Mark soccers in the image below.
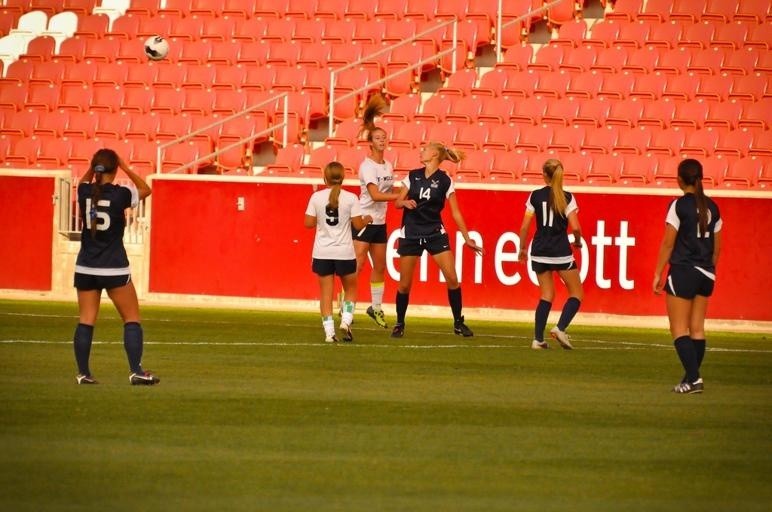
[144,36,171,61]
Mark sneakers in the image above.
[550,326,572,349]
[339,309,353,342]
[75,374,98,385]
[671,378,705,393]
[325,335,339,343]
[392,323,404,336]
[367,306,387,328]
[128,370,160,386]
[531,340,551,350]
[454,316,473,337]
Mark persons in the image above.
[392,141,486,337]
[338,127,400,328]
[652,159,723,395]
[518,159,583,351]
[72,148,160,385]
[302,162,373,342]
